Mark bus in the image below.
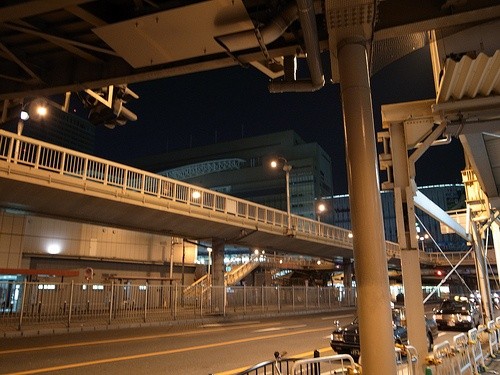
[331,272,357,288]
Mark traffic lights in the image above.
[436,268,443,276]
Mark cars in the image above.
[330,301,439,361]
[471,288,500,309]
[434,296,479,330]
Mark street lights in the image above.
[317,201,325,221]
[206,247,212,284]
[271,157,292,231]
[417,231,429,251]
[13,98,48,163]
